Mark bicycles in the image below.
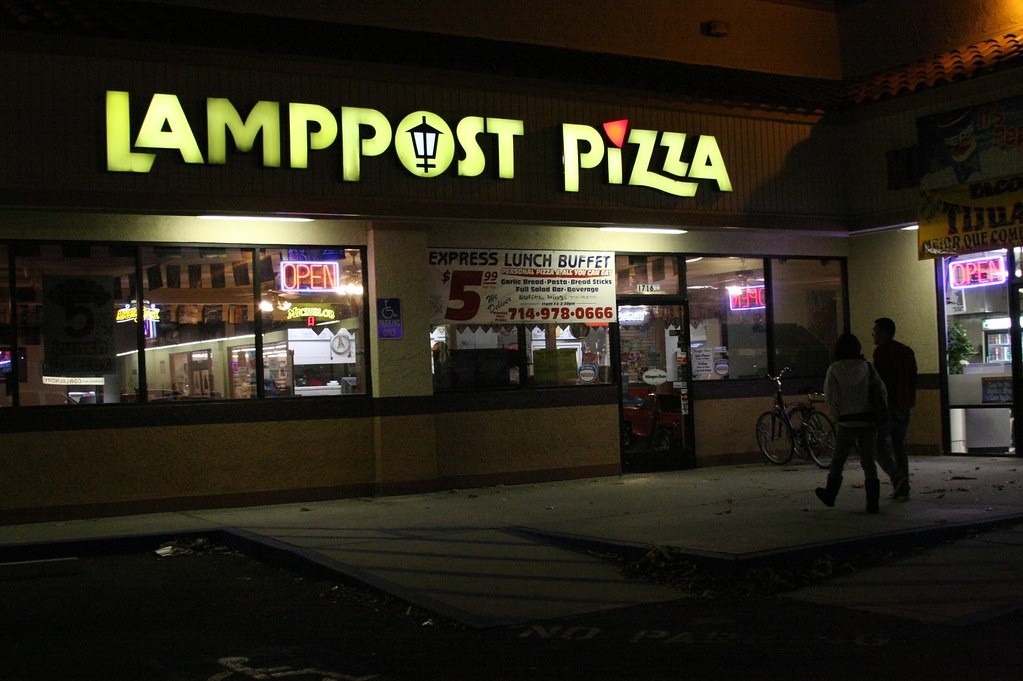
[753,366,859,470]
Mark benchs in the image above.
[625,407,681,453]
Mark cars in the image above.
[623,390,682,451]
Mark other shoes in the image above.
[889,486,912,501]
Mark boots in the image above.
[814,472,844,506]
[864,478,880,515]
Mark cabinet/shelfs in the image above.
[270,368,289,391]
[233,371,256,398]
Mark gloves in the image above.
[878,408,891,430]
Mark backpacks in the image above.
[828,359,876,405]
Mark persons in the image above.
[815,332,887,513]
[871,317,917,501]
